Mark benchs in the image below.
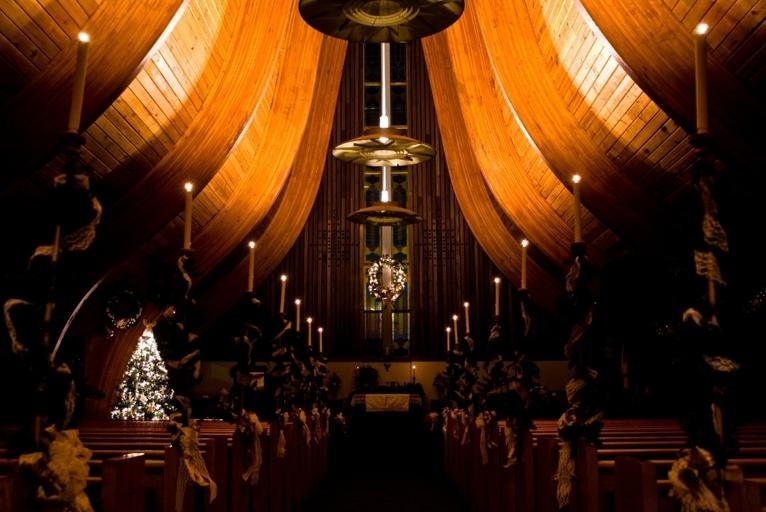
[0,407,330,512]
[441,408,766,511]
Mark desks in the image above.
[351,387,424,407]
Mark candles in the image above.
[279,274,324,351]
[184,183,192,248]
[522,238,529,287]
[493,277,502,316]
[247,240,255,292]
[444,301,470,352]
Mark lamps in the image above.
[297,0,467,229]
